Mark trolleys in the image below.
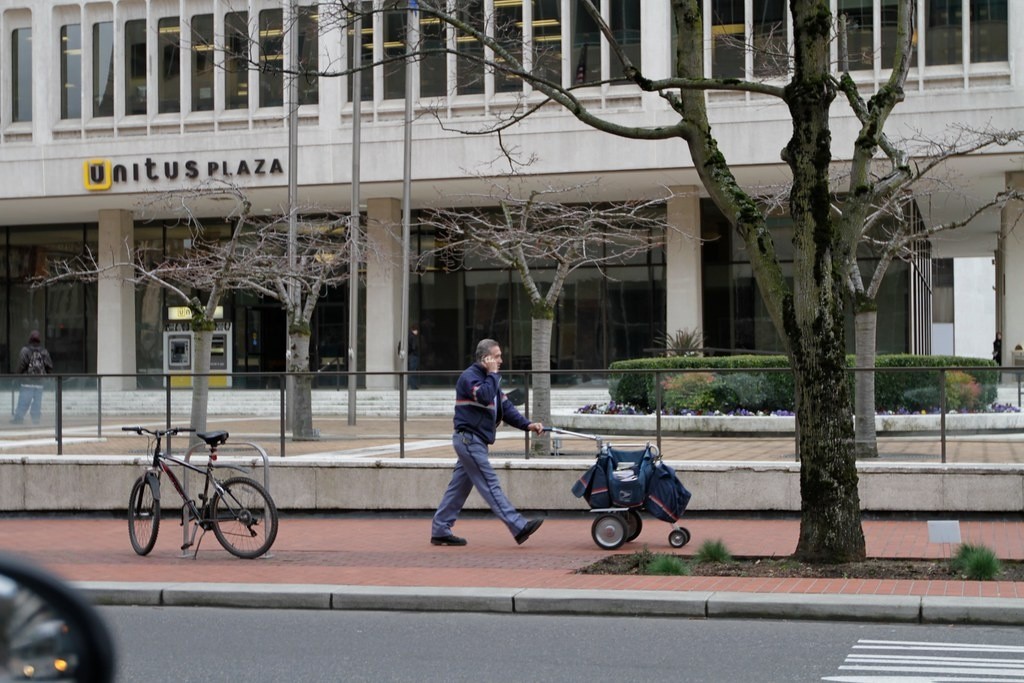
[543,424,692,551]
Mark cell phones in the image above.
[481,358,485,363]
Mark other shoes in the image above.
[33,421,40,425]
[9,418,23,424]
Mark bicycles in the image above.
[121,425,279,563]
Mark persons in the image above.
[992,332,1002,384]
[9,331,54,424]
[409,328,420,390]
[430,339,544,547]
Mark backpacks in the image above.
[24,345,48,380]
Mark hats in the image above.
[29,330,40,339]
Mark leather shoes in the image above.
[431,534,468,546]
[514,519,544,545]
